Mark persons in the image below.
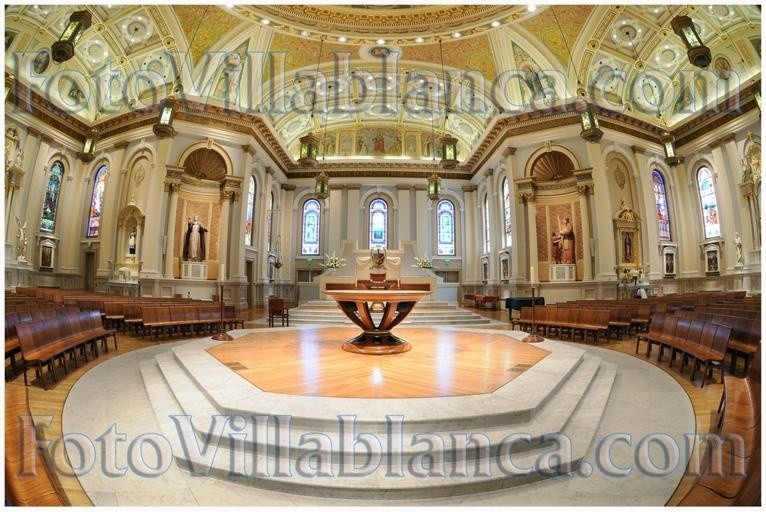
[551,231,563,264]
[356,130,405,156]
[187,215,209,262]
[127,225,138,254]
[560,216,575,263]
[624,232,632,260]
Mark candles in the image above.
[530,266,534,286]
[220,264,225,282]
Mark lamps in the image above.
[152,4,213,139]
[546,5,606,146]
[426,173,442,202]
[659,5,712,70]
[622,27,680,164]
[433,37,458,169]
[80,26,142,163]
[314,171,330,201]
[296,36,325,166]
[51,7,93,63]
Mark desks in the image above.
[464,294,500,310]
[320,281,431,354]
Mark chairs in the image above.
[266,297,290,327]
[510,285,761,507]
[6,288,244,506]
